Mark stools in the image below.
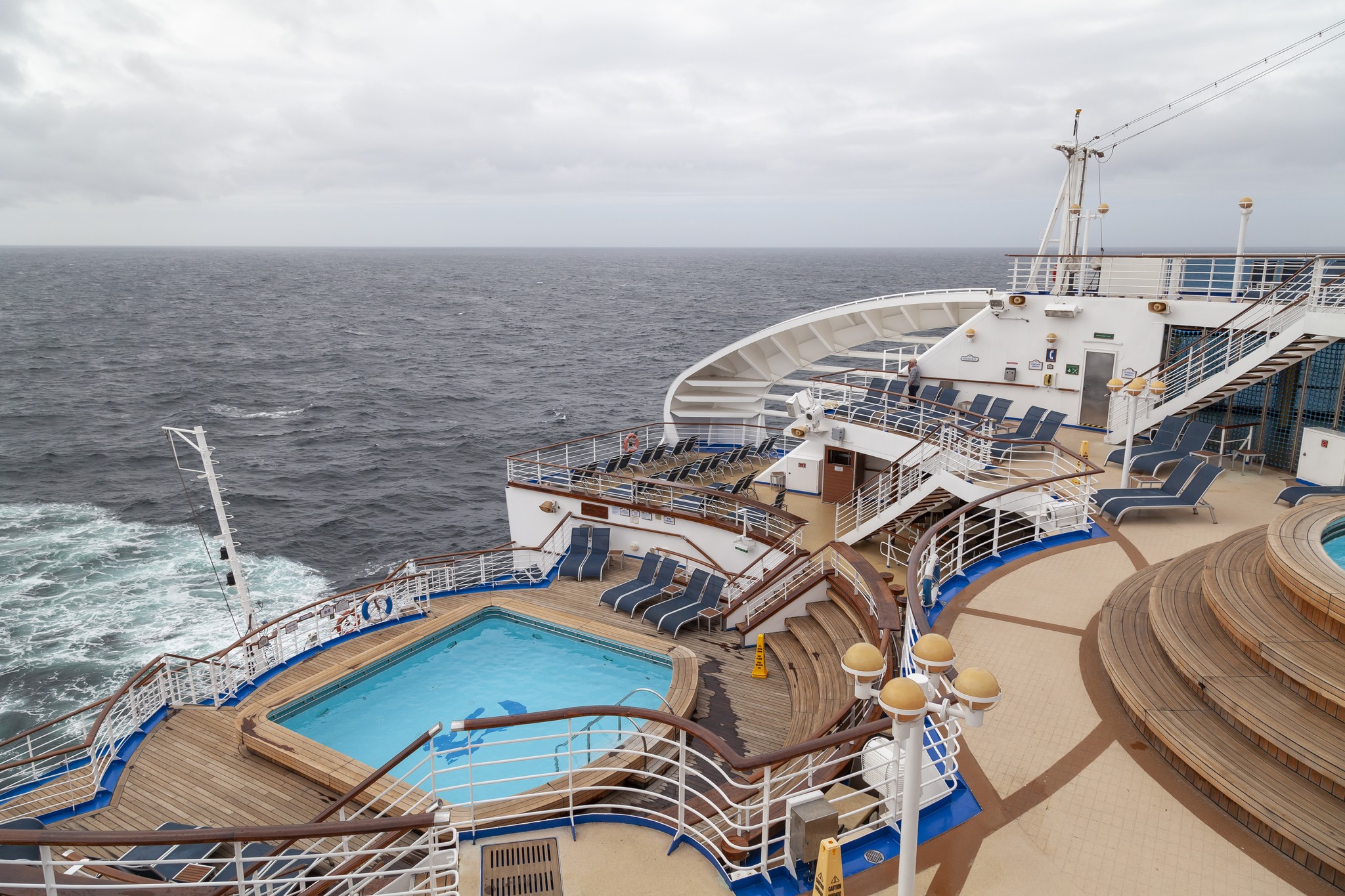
[720,836,748,878]
[888,584,905,613]
[896,597,907,642]
[879,572,894,587]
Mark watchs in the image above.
[908,384,911,386]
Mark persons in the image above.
[908,358,921,409]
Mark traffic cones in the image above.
[752,632,769,678]
[812,837,844,896]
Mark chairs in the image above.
[1273,486,1345,506]
[849,757,879,829]
[598,552,727,639]
[558,527,590,582]
[1089,415,1225,526]
[82,822,334,896]
[580,527,610,582]
[537,435,788,529]
[513,563,543,583]
[833,378,1069,464]
[1241,260,1345,306]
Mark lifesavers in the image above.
[361,593,393,624]
[1052,263,1058,283]
[624,434,639,454]
[336,610,360,636]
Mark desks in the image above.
[958,401,973,417]
[1231,449,1266,476]
[1130,476,1164,488]
[607,550,624,570]
[1189,450,1222,468]
[770,471,786,491]
[824,782,880,830]
[737,481,755,498]
[697,607,722,635]
[690,444,699,460]
[592,470,602,488]
[895,401,916,412]
[660,584,684,602]
[995,423,1019,434]
[172,863,216,883]
[769,502,788,512]
[660,452,669,468]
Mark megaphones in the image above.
[1008,295,1026,306]
[1148,300,1169,313]
